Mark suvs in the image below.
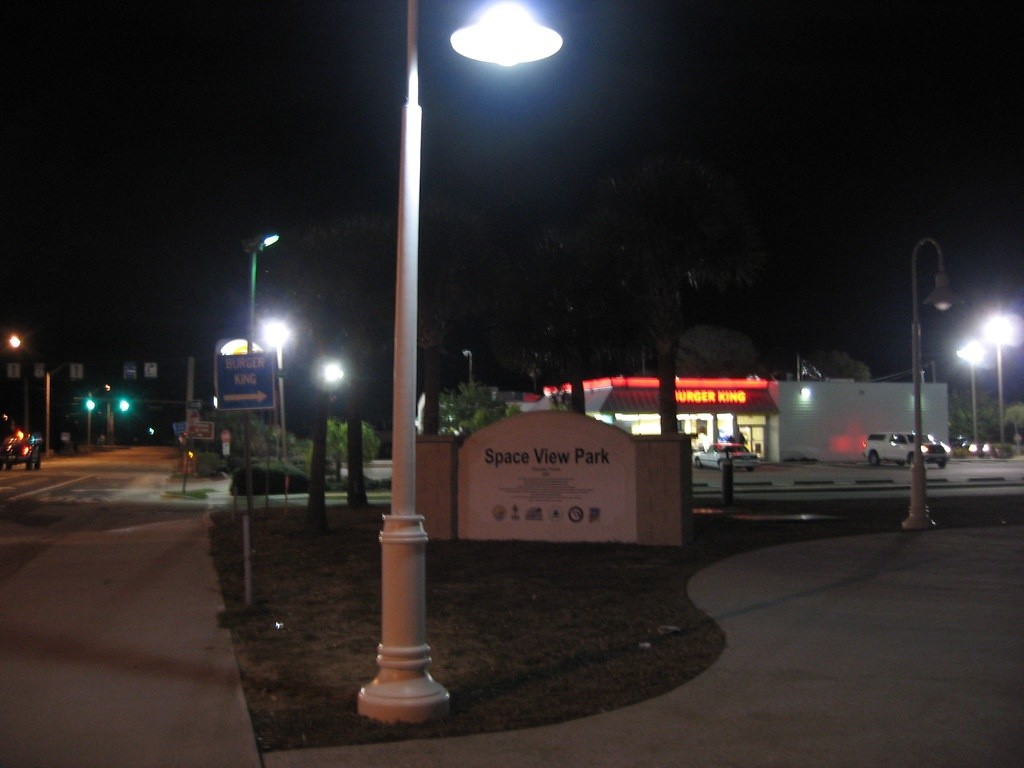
[862,432,954,468]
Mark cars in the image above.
[691,442,762,470]
[0,434,42,470]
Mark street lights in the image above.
[86,401,95,449]
[899,237,968,532]
[246,234,282,507]
[984,318,1011,445]
[962,342,985,448]
[463,350,474,386]
[354,0,562,725]
[10,335,29,432]
